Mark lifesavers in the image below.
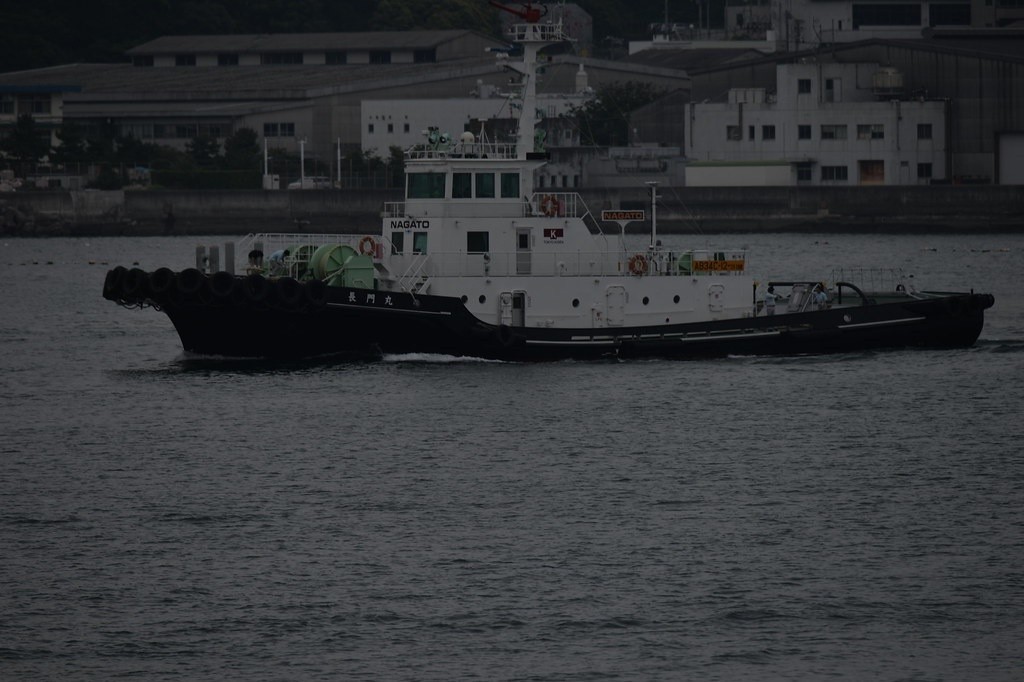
[359,236,376,256]
[631,253,647,272]
[104,266,325,310]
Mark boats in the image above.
[103,0,996,360]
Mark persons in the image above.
[814,282,827,311]
[765,287,778,316]
[249,249,290,276]
[459,127,475,153]
[541,194,560,218]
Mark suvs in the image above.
[287,176,331,191]
